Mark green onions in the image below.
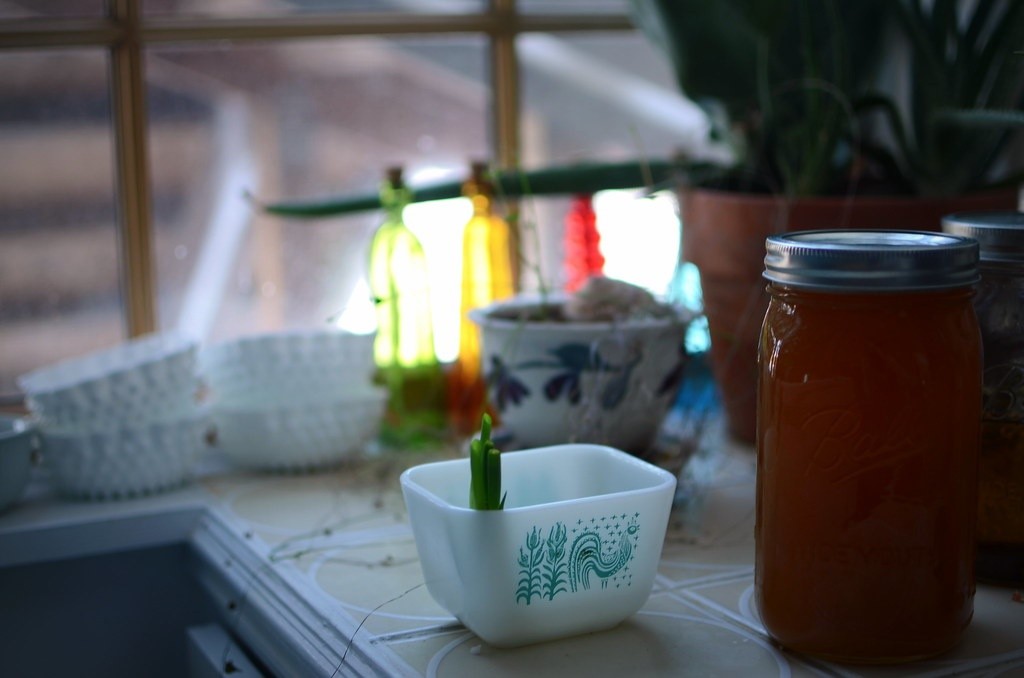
[468,412,507,510]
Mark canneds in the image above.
[752,211,1024,667]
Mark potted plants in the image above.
[623,1,1022,446]
[474,275,695,456]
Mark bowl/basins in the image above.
[0,415,33,516]
[400,442,678,649]
[19,327,387,501]
